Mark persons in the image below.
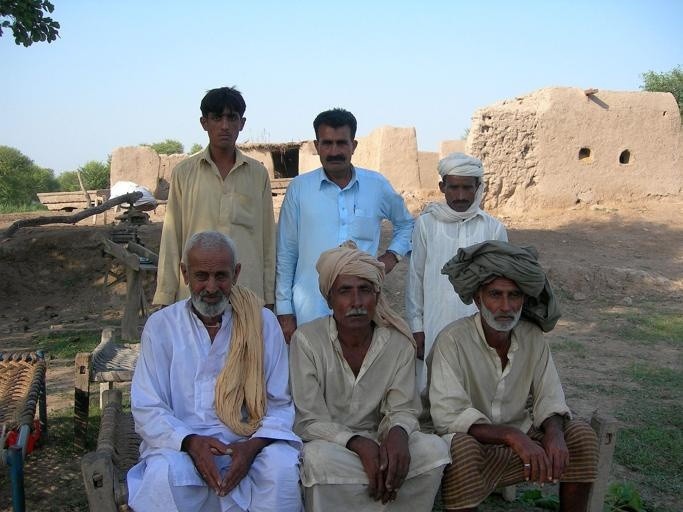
[275,109,414,345]
[407,152,508,361]
[289,240,448,512]
[131,230,302,511]
[429,241,599,512]
[152,88,277,314]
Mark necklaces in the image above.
[202,319,225,330]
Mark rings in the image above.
[523,463,531,467]
